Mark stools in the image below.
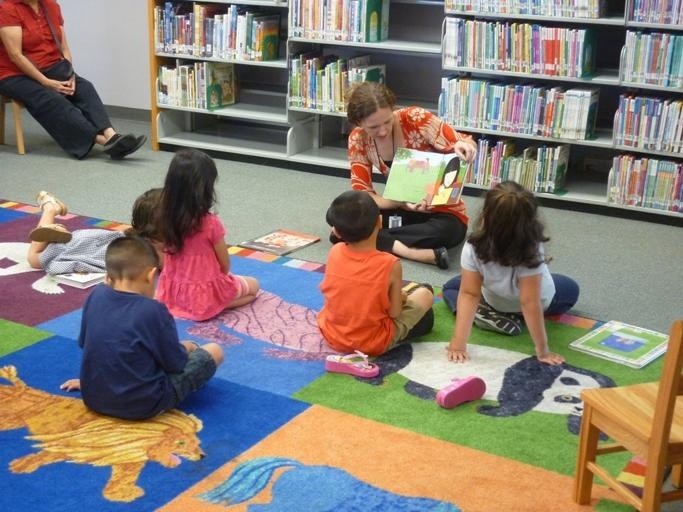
[0,96,29,155]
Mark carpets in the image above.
[0,200,682,512]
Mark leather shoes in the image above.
[330,230,339,246]
[432,250,449,268]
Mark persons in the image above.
[60,236,224,421]
[327,82,479,270]
[317,191,434,356]
[0,0,148,160]
[27,188,167,277]
[442,181,579,367]
[153,149,259,322]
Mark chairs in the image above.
[574,321,683,512]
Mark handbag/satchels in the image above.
[41,4,76,80]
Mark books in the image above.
[464,137,569,194]
[287,0,390,44]
[443,16,598,79]
[238,228,321,257]
[441,73,602,141]
[622,29,682,88]
[155,59,238,110]
[625,1,683,26]
[609,151,683,213]
[443,1,609,19]
[617,91,683,154]
[52,273,112,289]
[287,51,386,113]
[155,1,281,61]
[383,148,472,206]
[569,320,670,369]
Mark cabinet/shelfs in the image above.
[149,2,683,220]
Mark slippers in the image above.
[432,377,486,409]
[323,351,381,377]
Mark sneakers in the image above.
[104,131,135,155]
[134,137,145,154]
[470,307,519,335]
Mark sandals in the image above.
[38,192,69,218]
[29,226,70,243]
